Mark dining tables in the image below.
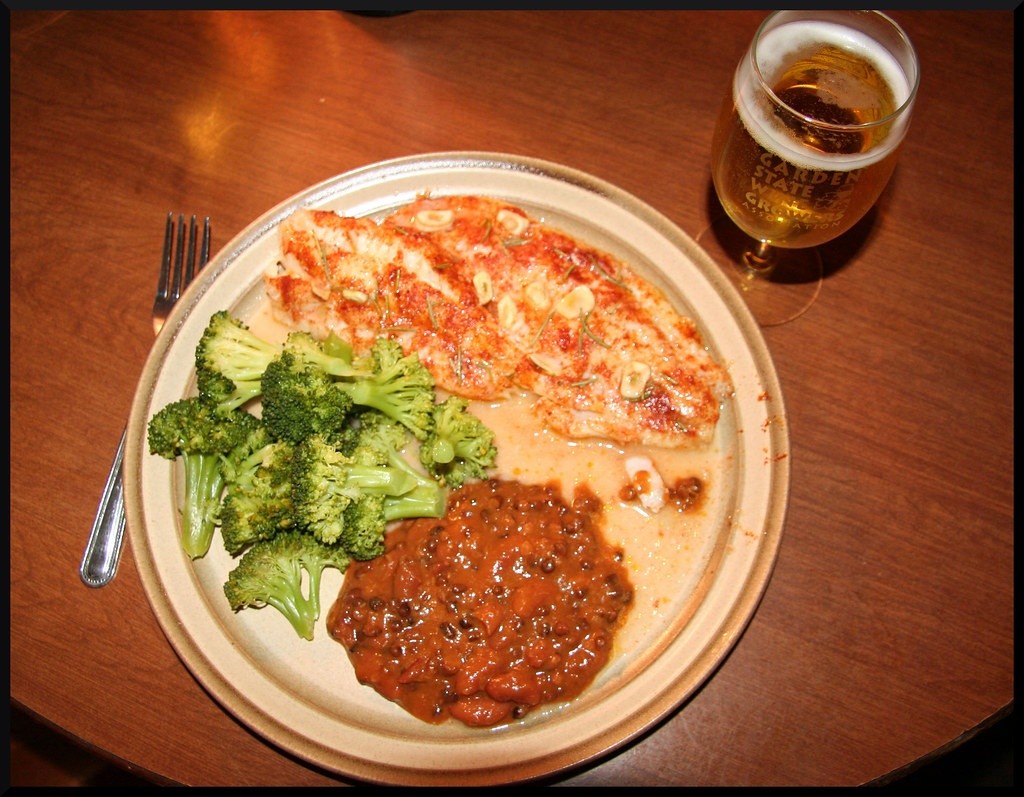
[9,10,1013,795]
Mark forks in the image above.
[78,210,212,588]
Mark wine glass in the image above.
[694,10,920,327]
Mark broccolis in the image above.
[149,314,491,638]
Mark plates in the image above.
[121,151,790,787]
